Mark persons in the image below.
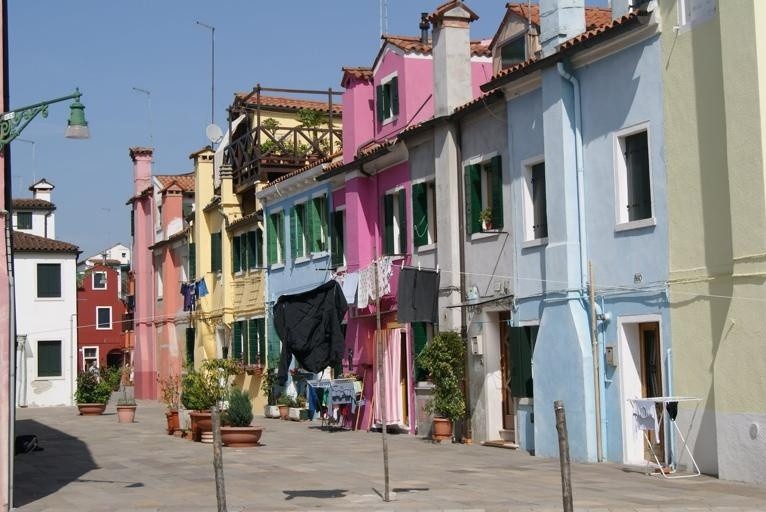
[89,361,100,383]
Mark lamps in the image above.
[1,84,88,153]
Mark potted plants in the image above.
[72,355,139,423]
[411,330,469,436]
[154,351,309,447]
